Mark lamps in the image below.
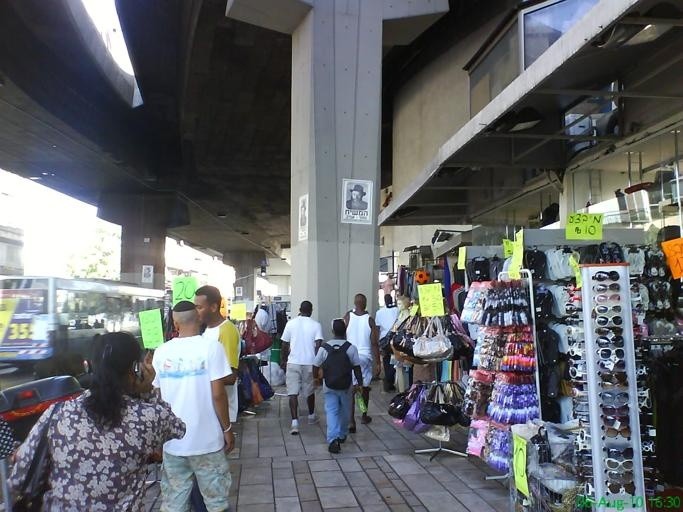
[391,206,419,218]
[597,23,674,49]
[437,167,482,177]
[485,107,542,133]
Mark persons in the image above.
[194,285,241,424]
[4,332,186,512]
[151,300,235,512]
[279,291,400,454]
[299,201,307,226]
[346,184,368,210]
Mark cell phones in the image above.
[133,360,145,381]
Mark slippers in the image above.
[536,283,574,399]
[525,247,580,280]
[631,279,677,335]
[582,241,671,279]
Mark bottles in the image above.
[356,392,367,413]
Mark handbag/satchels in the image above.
[388,383,471,442]
[239,372,276,412]
[246,330,274,356]
[377,327,475,365]
[11,439,54,512]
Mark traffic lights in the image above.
[260,265,266,277]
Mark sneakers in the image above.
[329,420,356,453]
[361,416,371,423]
[290,426,299,434]
[308,416,321,425]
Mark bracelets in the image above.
[223,425,232,434]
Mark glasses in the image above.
[563,271,656,496]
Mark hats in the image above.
[350,185,366,196]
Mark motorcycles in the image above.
[0,353,96,443]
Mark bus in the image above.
[0,274,170,366]
[259,295,290,321]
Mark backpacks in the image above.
[320,341,352,390]
